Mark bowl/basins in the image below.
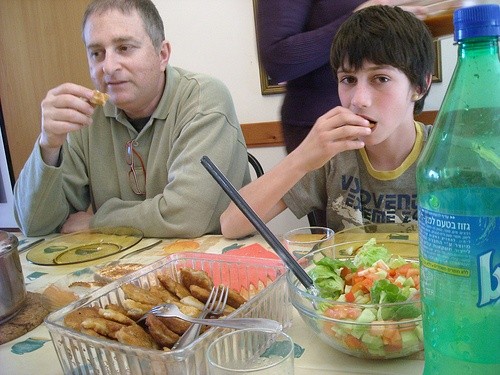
[285,241,426,358]
[46,251,289,375]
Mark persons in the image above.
[257,0,463,234]
[220,4,500,238]
[13,0,253,238]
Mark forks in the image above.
[171,281,230,358]
[134,304,283,334]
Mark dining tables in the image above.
[0,232,425,375]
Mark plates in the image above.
[26,227,145,266]
[332,220,419,256]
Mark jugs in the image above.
[0,231,26,325]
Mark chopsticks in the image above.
[198,156,315,292]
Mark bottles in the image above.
[417,5,500,375]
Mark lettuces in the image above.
[305,234,423,324]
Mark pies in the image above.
[60,266,250,354]
[88,90,110,106]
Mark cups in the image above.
[205,328,294,375]
[285,227,333,259]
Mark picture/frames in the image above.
[253,0,443,96]
